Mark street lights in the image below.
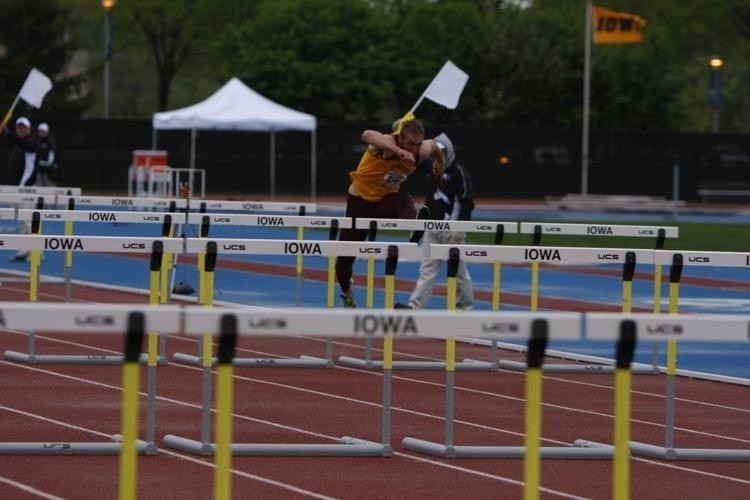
[101,0,118,118]
[709,54,725,131]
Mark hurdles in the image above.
[337,218,519,371]
[628,248,750,461]
[183,304,582,500]
[163,234,421,457]
[55,194,187,307]
[188,199,318,306]
[3,208,188,367]
[0,300,182,500]
[2,232,184,455]
[174,212,353,369]
[0,186,82,284]
[402,243,656,459]
[500,220,679,376]
[584,310,750,500]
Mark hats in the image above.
[37,122,49,132]
[15,116,30,127]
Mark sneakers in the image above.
[409,206,431,244]
[393,302,417,312]
[340,278,357,309]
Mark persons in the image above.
[5,117,39,263]
[391,132,475,314]
[36,123,60,186]
[333,118,445,308]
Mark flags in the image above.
[19,66,54,109]
[591,8,648,46]
[423,62,470,110]
